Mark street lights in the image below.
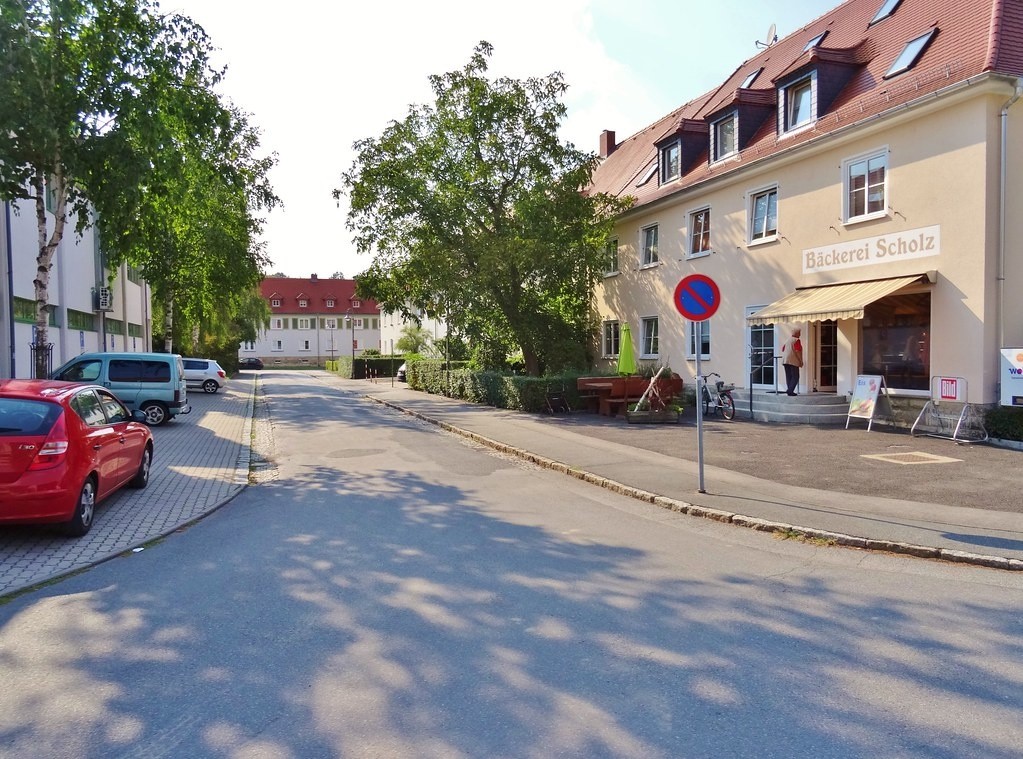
[344,308,355,378]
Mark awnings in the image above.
[746,273,926,326]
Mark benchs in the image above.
[577,377,683,418]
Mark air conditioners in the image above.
[99,286,112,310]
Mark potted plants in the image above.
[626,400,685,425]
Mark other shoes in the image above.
[786,390,797,396]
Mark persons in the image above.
[782,328,803,396]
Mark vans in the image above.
[46,353,188,426]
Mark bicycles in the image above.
[695,372,736,420]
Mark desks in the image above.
[584,382,613,415]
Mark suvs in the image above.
[181,358,227,394]
[239,358,264,370]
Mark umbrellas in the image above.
[615,320,636,417]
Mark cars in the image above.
[0,379,154,534]
[397,364,406,382]
